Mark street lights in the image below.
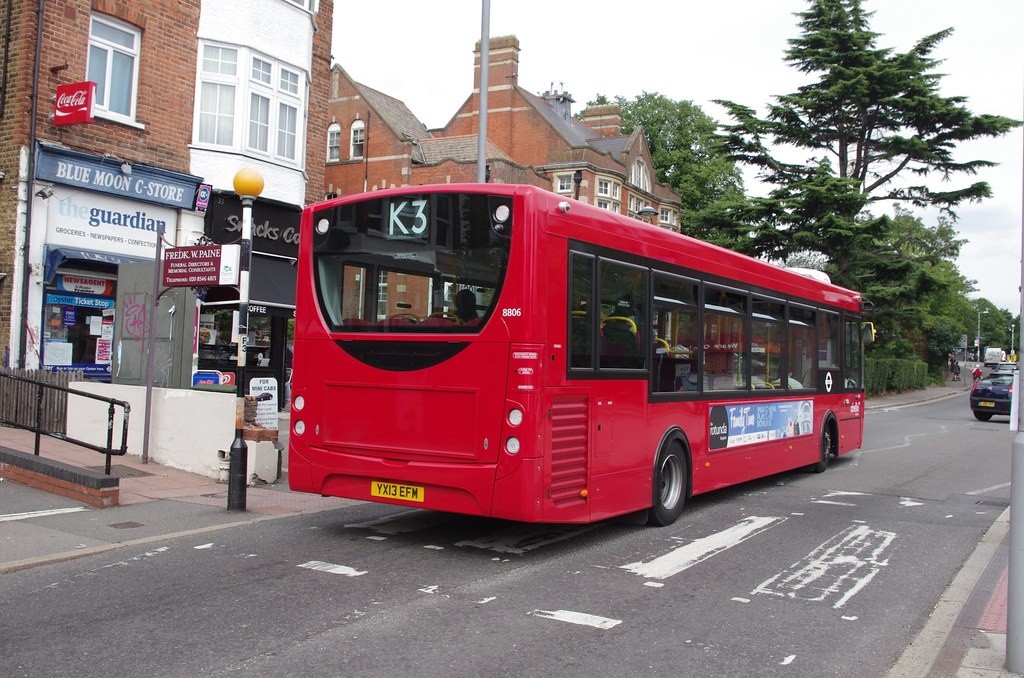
[977,311,989,366]
[217,162,266,513]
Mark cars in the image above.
[968,372,1014,421]
[992,362,1019,373]
[734,351,767,377]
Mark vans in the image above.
[984,347,1002,368]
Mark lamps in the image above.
[35,185,54,199]
[121,160,133,176]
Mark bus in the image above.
[287,180,878,528]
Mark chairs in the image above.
[344,309,482,329]
[573,310,792,389]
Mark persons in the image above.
[453,289,479,322]
[952,362,961,381]
[600,294,655,345]
[972,365,983,382]
[950,357,956,368]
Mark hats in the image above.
[976,365,979,368]
